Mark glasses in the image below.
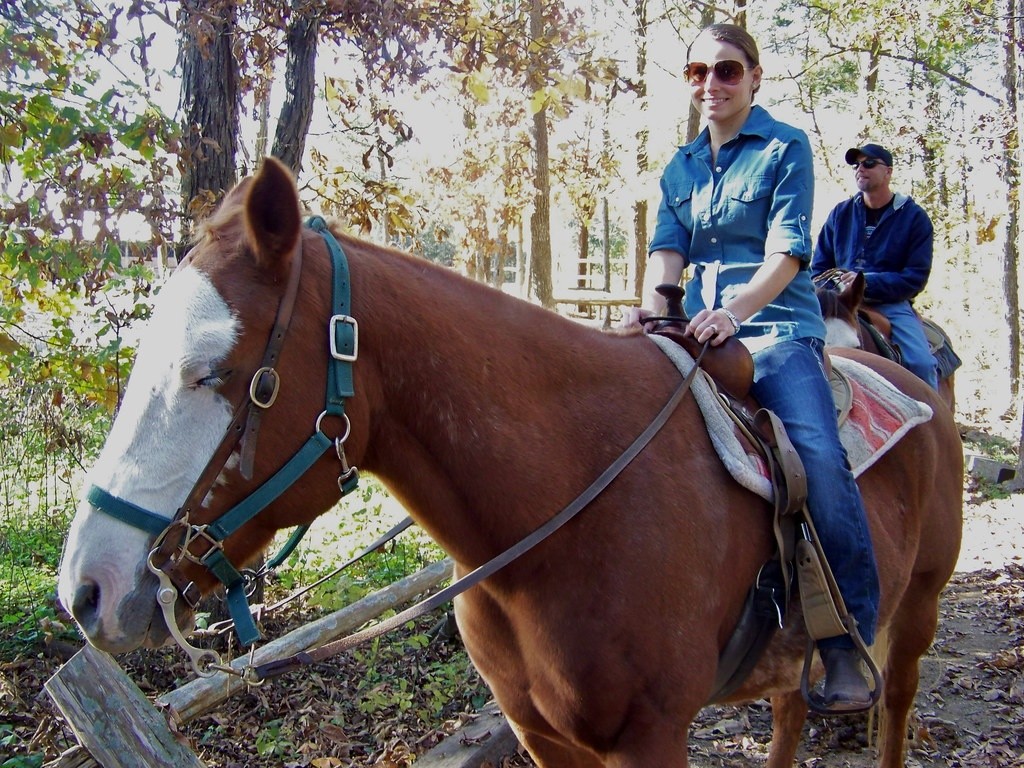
[851,159,888,170]
[684,60,755,86]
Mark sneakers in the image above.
[818,644,869,711]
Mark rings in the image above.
[710,324,717,334]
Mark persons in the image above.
[811,144,939,392]
[619,24,880,710]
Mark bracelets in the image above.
[719,309,739,333]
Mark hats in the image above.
[844,143,893,166]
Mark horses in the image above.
[58,157,965,768]
